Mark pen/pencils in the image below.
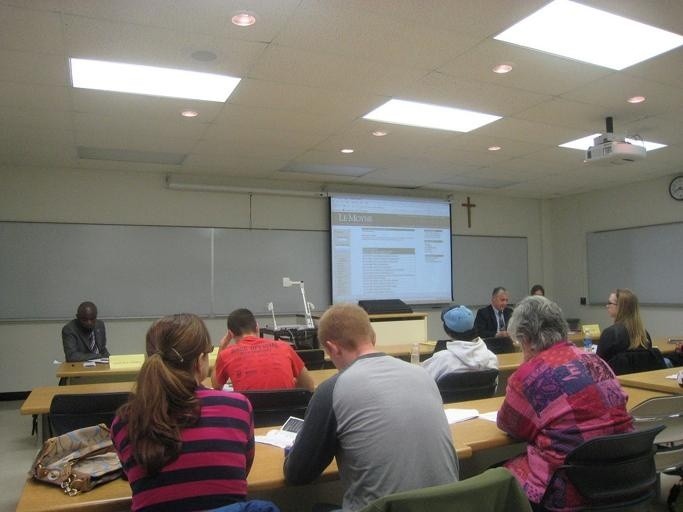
[72,364,82,367]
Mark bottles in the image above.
[582,329,594,352]
[410,342,420,364]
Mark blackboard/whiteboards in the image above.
[586,219,683,305]
[1,221,528,322]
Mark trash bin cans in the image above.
[656,466,683,512]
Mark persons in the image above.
[109,313,254,511]
[495,295,638,512]
[530,285,545,296]
[594,288,651,375]
[61,302,109,362]
[421,304,500,403]
[660,342,682,367]
[281,302,459,511]
[212,308,315,426]
[475,287,515,336]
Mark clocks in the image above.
[667,174,682,201]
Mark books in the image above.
[83,361,95,367]
[254,416,304,448]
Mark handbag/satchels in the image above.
[31,423,124,498]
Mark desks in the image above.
[14,329,680,512]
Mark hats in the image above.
[441,305,475,333]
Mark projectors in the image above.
[582,141,647,169]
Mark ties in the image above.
[87,332,97,354]
[498,311,505,331]
